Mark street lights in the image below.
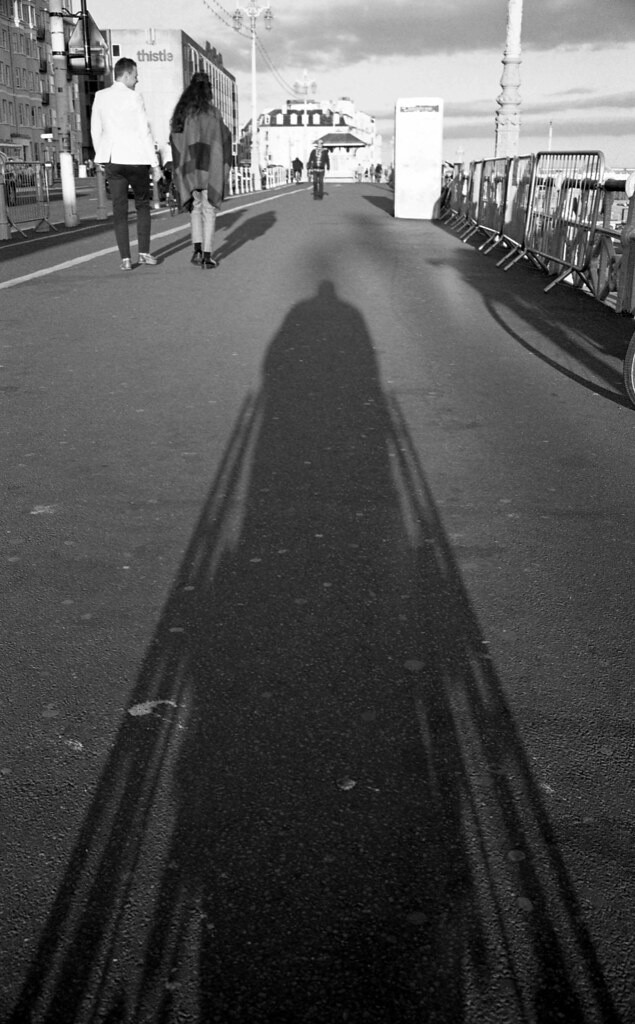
[292,79,319,182]
[232,1,276,192]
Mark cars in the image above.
[11,156,36,187]
[0,151,18,207]
[104,153,172,201]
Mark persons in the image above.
[308,139,330,200]
[292,157,303,181]
[90,57,164,269]
[167,72,231,269]
[355,163,385,184]
[84,158,95,177]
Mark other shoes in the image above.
[119,258,133,271]
[191,252,202,266]
[136,252,157,265]
[202,252,218,268]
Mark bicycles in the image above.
[295,168,304,184]
[165,168,179,217]
